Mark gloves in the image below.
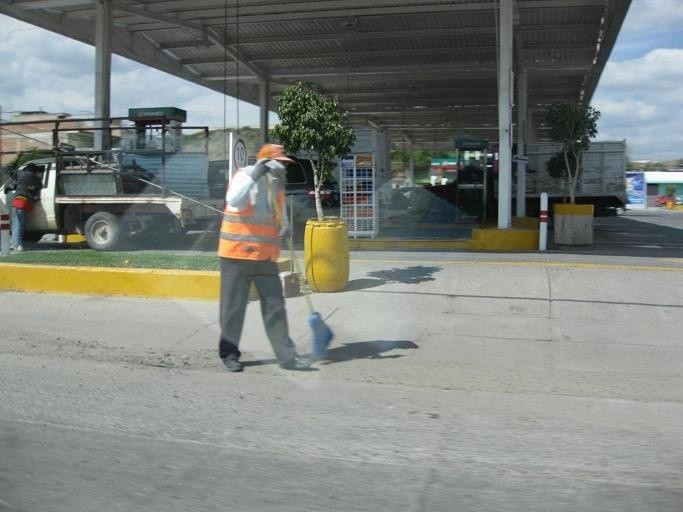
[251,165,268,180]
[279,226,292,238]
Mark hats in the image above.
[256,143,295,164]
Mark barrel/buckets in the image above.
[305,215,349,292]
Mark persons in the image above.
[215,144,311,373]
[7,163,42,252]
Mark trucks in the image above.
[409,138,629,224]
[0,114,225,253]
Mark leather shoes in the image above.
[282,354,311,369]
[223,356,243,372]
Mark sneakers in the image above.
[14,245,24,252]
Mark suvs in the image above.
[207,154,342,245]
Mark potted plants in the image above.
[537,101,602,247]
[266,82,360,294]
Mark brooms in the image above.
[261,172,335,360]
